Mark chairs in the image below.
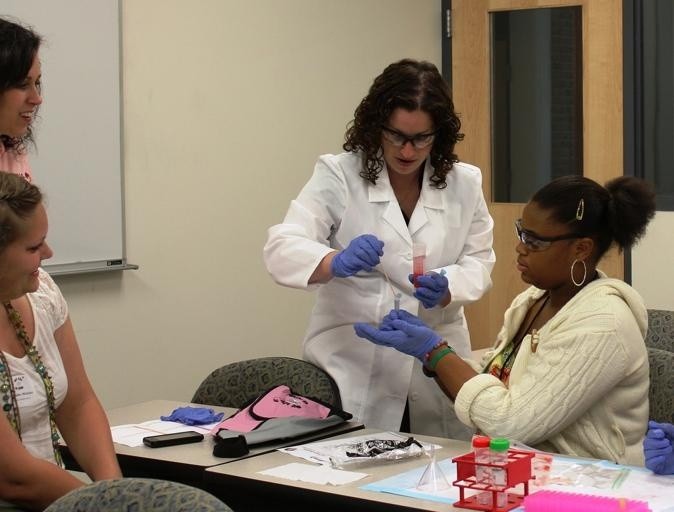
[646,309,673,422]
[189,356,342,409]
[43,477,231,511]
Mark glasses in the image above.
[514,219,592,252]
[381,125,438,149]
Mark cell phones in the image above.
[143,431,204,448]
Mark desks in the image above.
[58,398,367,488]
[204,425,674,511]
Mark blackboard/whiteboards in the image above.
[1,0,138,279]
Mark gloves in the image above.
[353,319,445,363]
[159,406,223,427]
[406,270,451,311]
[379,309,431,334]
[331,234,385,279]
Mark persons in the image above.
[261,57,496,436]
[352,172,657,469]
[0,14,43,187]
[0,170,125,512]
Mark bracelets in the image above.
[429,348,453,368]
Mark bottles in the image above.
[490,437,510,509]
[394,298,400,316]
[412,241,425,290]
[438,269,447,278]
[473,433,490,507]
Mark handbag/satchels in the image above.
[211,383,354,445]
